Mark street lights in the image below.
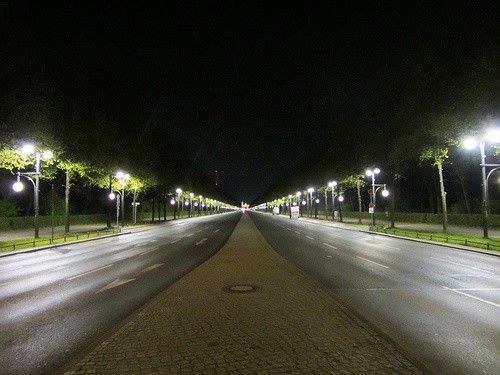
[463,126,500,236]
[327,180,343,220]
[307,187,320,218]
[13,142,53,238]
[108,172,131,226]
[366,168,389,225]
[171,188,236,218]
[251,191,307,219]
